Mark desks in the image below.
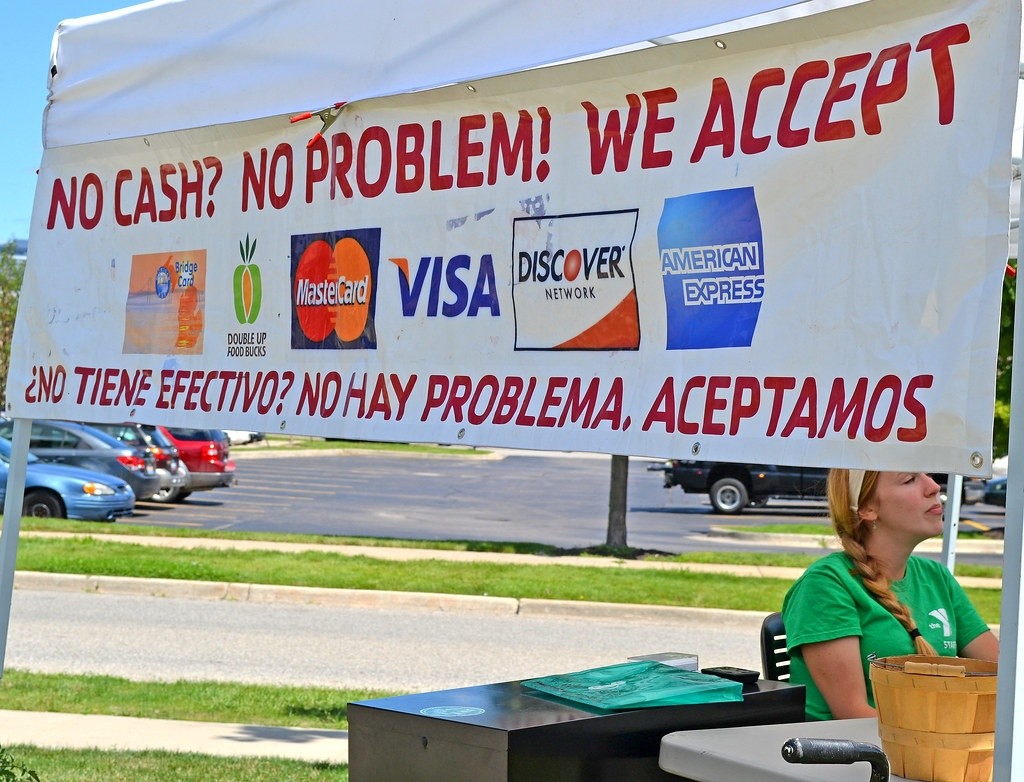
[658,718,925,782]
[346,674,805,782]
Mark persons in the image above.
[781,469,999,725]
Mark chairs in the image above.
[761,611,791,682]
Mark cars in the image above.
[647,459,971,516]
[964,476,1007,508]
[162,427,234,500]
[0,434,137,523]
[0,411,161,503]
[68,419,187,504]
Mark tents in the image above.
[0,0,1024,782]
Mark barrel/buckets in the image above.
[866,651,998,782]
